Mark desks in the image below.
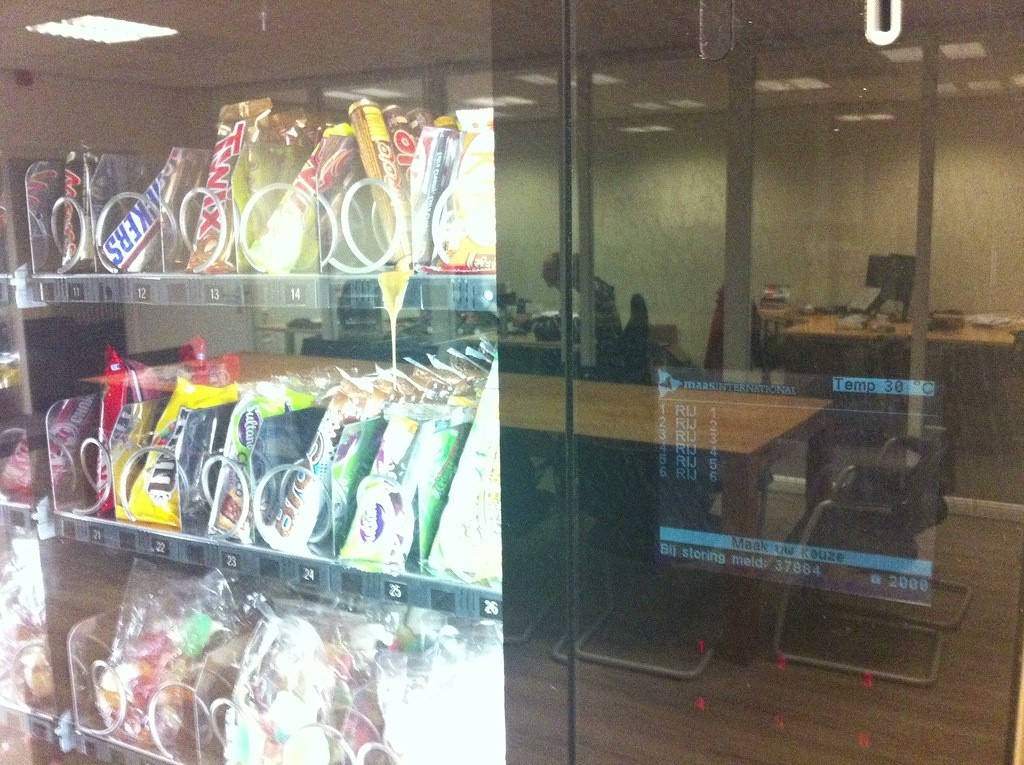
[780,311,1024,349]
[457,325,582,354]
[78,353,836,663]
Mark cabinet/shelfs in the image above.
[16,264,507,764]
[254,322,322,354]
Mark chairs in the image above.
[551,433,730,683]
[768,424,977,689]
[623,292,650,356]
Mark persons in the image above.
[512,250,621,368]
